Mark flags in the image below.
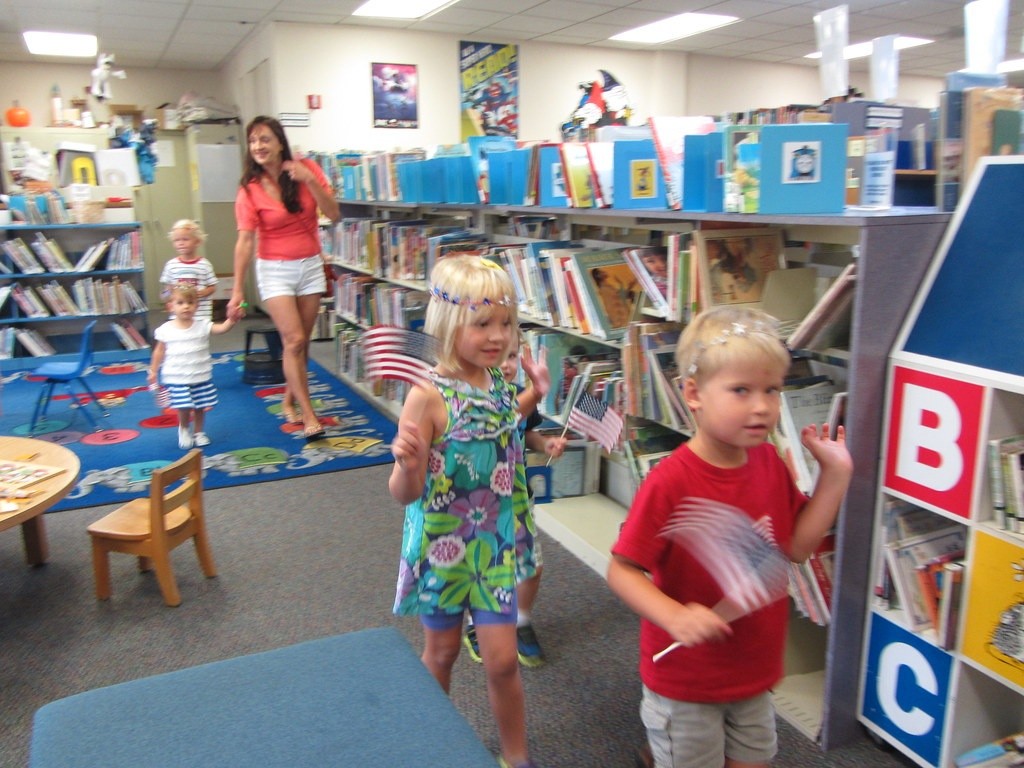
[361,323,438,395]
[566,388,624,455]
[149,383,171,409]
[655,494,794,625]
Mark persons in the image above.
[607,302,854,768]
[226,115,340,438]
[591,267,638,328]
[148,219,246,449]
[388,254,568,768]
[635,247,668,299]
[710,234,757,283]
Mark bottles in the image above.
[50,87,62,125]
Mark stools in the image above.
[243,322,292,386]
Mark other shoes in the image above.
[177,427,194,450]
[192,431,211,447]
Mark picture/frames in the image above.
[372,62,420,129]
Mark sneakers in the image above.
[514,622,548,668]
[462,624,484,663]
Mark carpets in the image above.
[0,343,402,512]
[29,625,496,768]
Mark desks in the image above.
[0,433,81,565]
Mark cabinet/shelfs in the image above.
[1,179,148,353]
[298,143,956,755]
[855,352,1024,768]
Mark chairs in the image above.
[31,323,106,427]
[88,449,217,606]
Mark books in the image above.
[952,731,1024,768]
[306,104,1024,650]
[0,195,158,360]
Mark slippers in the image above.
[302,418,325,439]
[281,403,302,424]
